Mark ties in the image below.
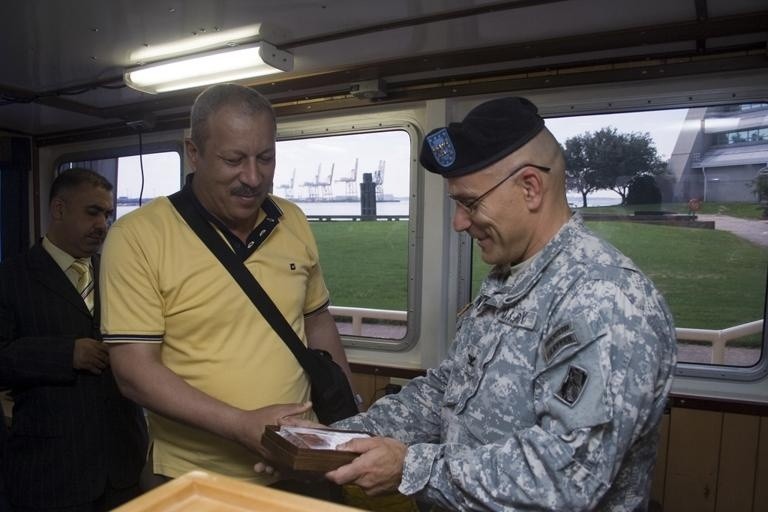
[70,257,96,320]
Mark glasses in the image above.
[444,163,551,214]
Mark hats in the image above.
[420,95,547,181]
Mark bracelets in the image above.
[352,393,362,407]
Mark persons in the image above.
[99,83,367,505]
[0,169,148,510]
[255,95,677,510]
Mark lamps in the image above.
[122,42,295,96]
[127,24,288,66]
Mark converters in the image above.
[385,384,401,396]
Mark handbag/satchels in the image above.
[303,344,360,431]
